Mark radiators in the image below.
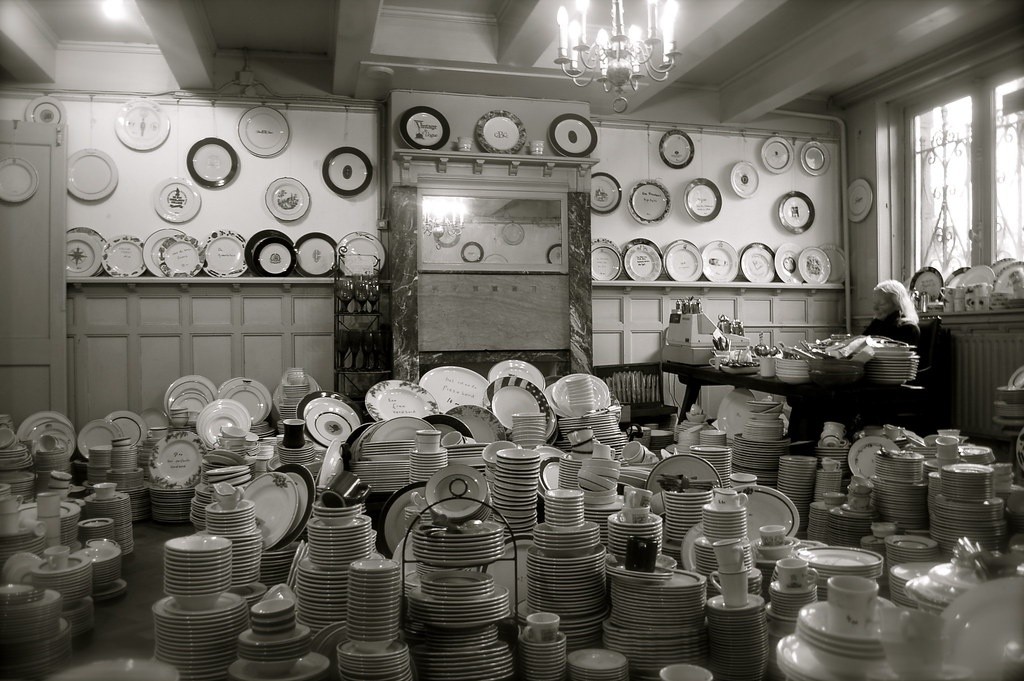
[954,332,1024,440]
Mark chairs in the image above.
[874,317,943,439]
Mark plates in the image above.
[1,96,1024,680]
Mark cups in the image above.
[250,599,294,639]
[213,485,244,510]
[525,613,559,643]
[283,420,307,448]
[566,427,595,444]
[641,447,657,464]
[824,458,840,470]
[592,442,615,458]
[710,488,748,509]
[622,485,653,507]
[321,471,372,505]
[529,142,545,156]
[408,492,430,509]
[407,429,443,453]
[42,546,73,572]
[825,577,880,636]
[715,540,744,571]
[706,569,752,606]
[617,508,654,524]
[626,534,660,571]
[760,526,787,546]
[820,429,848,448]
[456,137,472,151]
[146,426,167,439]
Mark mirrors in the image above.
[416,186,570,351]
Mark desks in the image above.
[662,360,902,459]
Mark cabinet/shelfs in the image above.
[334,252,393,400]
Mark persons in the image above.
[862,280,927,411]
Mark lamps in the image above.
[422,195,466,250]
[552,0,683,114]
